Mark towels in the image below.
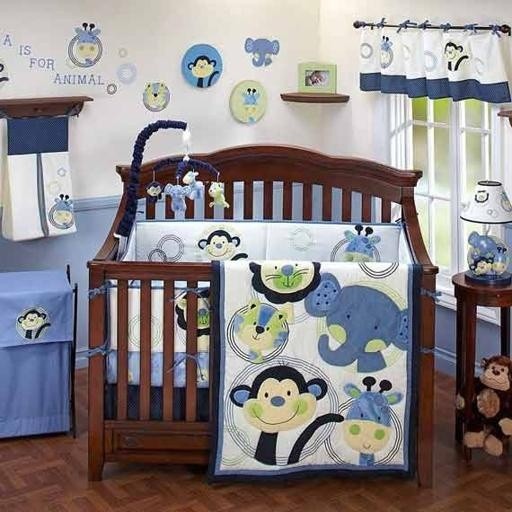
[0,116,77,243]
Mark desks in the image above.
[451,269,512,460]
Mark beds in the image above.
[84,143,438,490]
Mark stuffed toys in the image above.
[165,183,191,214]
[208,181,231,210]
[145,181,164,204]
[456,355,512,455]
[182,170,205,201]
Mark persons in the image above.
[304,70,327,87]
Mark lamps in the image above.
[457,178,512,287]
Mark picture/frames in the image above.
[295,61,339,95]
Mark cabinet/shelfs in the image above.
[0,263,80,445]
